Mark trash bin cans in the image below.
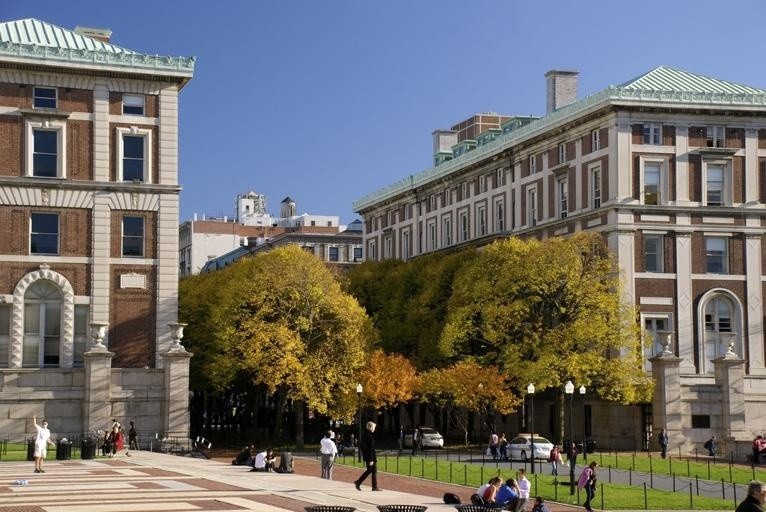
[56,439,72,459]
[81,437,96,459]
[587,440,596,453]
[27,440,36,461]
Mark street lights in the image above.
[564,379,578,494]
[527,382,538,474]
[356,381,364,463]
[578,381,588,460]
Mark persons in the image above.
[708,435,716,457]
[567,442,578,465]
[354,422,383,491]
[328,429,356,455]
[129,421,139,450]
[658,428,668,460]
[550,445,560,475]
[109,419,124,454]
[320,432,337,480]
[398,425,424,456]
[753,435,766,465]
[577,461,597,512]
[236,442,295,473]
[32,412,56,474]
[478,468,549,512]
[487,430,507,460]
[735,480,766,512]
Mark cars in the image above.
[507,433,558,462]
[397,425,446,449]
